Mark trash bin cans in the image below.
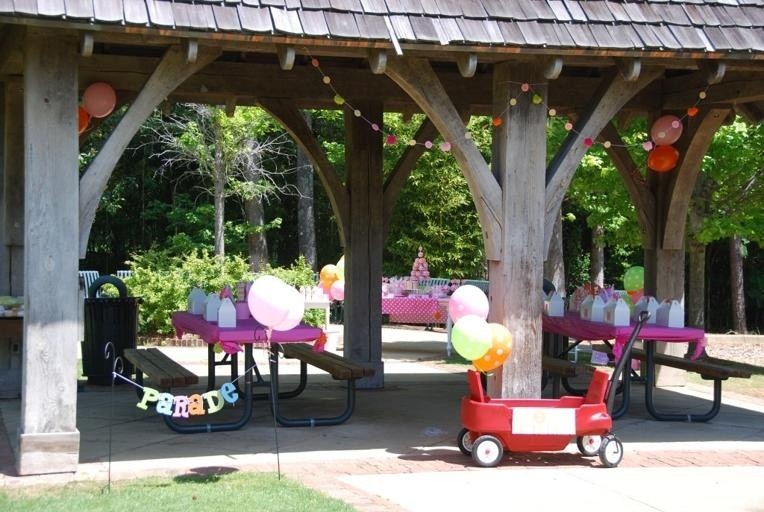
[84,296,144,386]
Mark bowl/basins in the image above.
[401,289,411,295]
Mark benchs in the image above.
[592,343,764,379]
[542,353,597,379]
[123,348,199,387]
[284,343,376,380]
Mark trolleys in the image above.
[443,309,654,467]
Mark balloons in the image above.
[272,284,305,333]
[647,146,680,173]
[82,82,116,118]
[79,105,90,135]
[446,285,489,324]
[317,255,344,301]
[651,116,683,146]
[247,276,291,328]
[450,315,492,361]
[624,266,644,291]
[473,322,514,373]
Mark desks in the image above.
[300,299,332,331]
[381,293,444,332]
[542,310,721,422]
[136,311,356,433]
